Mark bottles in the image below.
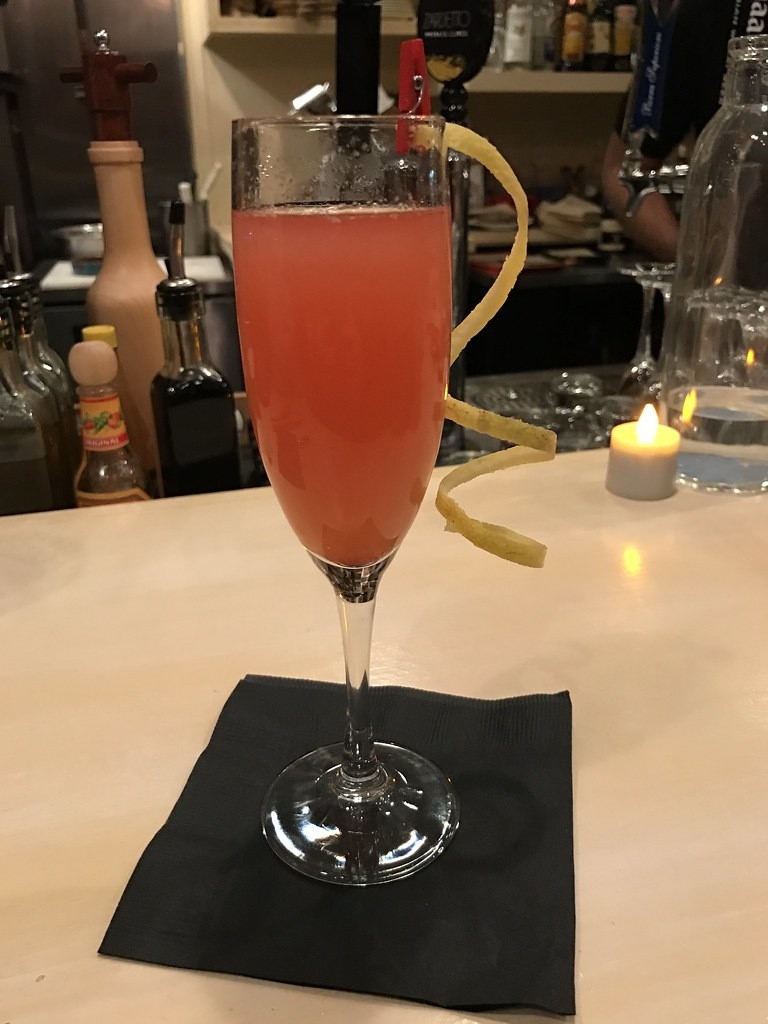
[81,140,172,464]
[663,32,768,495]
[71,384,152,510]
[0,269,70,514]
[147,276,239,499]
[492,0,634,73]
[79,325,160,498]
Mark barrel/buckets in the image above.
[156,198,210,256]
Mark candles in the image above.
[606,404,681,500]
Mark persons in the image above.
[601,0,768,262]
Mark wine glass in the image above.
[229,112,463,889]
[608,261,675,397]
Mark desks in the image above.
[0,448,768,1024]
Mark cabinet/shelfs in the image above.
[180,1,646,445]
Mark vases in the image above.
[84,139,175,479]
